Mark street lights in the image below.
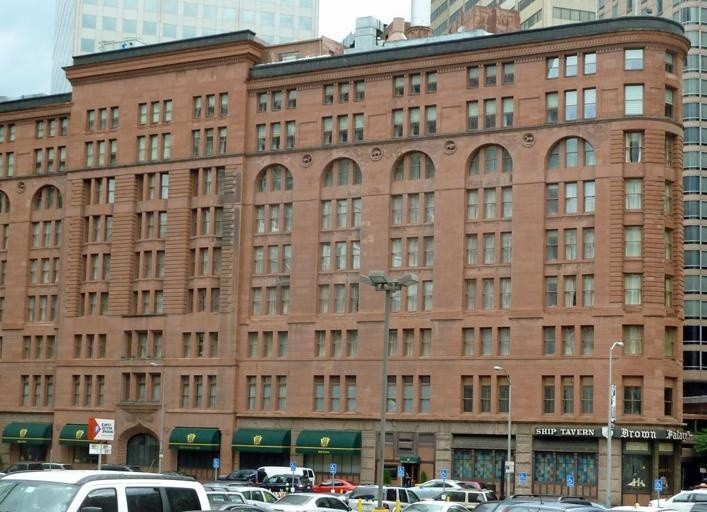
[149,361,167,475]
[493,365,515,499]
[606,340,625,511]
[358,269,419,512]
